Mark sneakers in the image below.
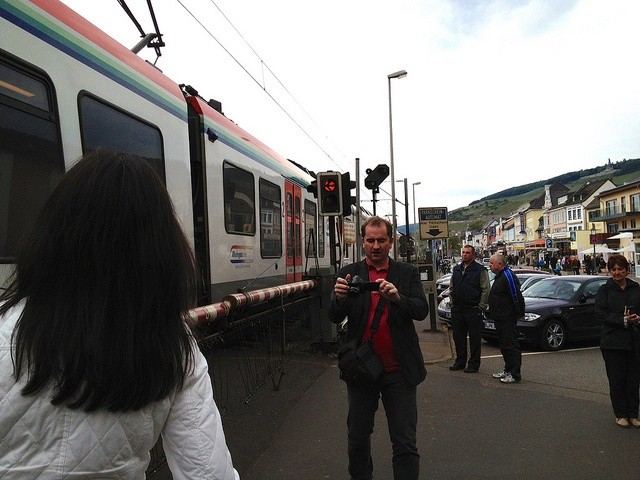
[492,369,511,378]
[628,416,640,427]
[499,374,519,384]
[615,417,629,427]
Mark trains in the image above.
[1,1,419,351]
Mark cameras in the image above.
[343,275,380,299]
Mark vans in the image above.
[482,258,491,268]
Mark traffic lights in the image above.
[317,172,343,216]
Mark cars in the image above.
[438,281,450,291]
[483,275,614,351]
[437,273,554,327]
[438,269,552,318]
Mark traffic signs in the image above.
[418,208,449,241]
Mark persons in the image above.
[435,257,440,272]
[327,217,429,480]
[448,244,489,373]
[0,146,241,480]
[530,252,606,275]
[504,253,520,267]
[488,253,526,383]
[594,255,640,427]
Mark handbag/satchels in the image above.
[337,336,385,391]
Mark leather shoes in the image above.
[449,363,465,370]
[464,367,478,373]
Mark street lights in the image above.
[387,70,409,269]
